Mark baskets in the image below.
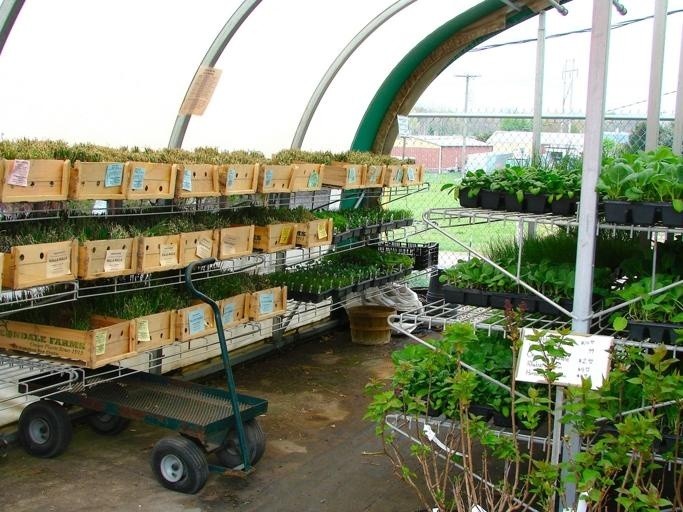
[367,241,439,271]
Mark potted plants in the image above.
[0,136,425,204]
[0,245,415,371]
[0,205,419,291]
[362,129,683,512]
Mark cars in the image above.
[447,165,460,173]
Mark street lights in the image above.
[561,68,579,133]
[453,72,482,168]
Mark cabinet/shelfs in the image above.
[0,180,438,466]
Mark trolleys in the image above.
[18,255,268,494]
